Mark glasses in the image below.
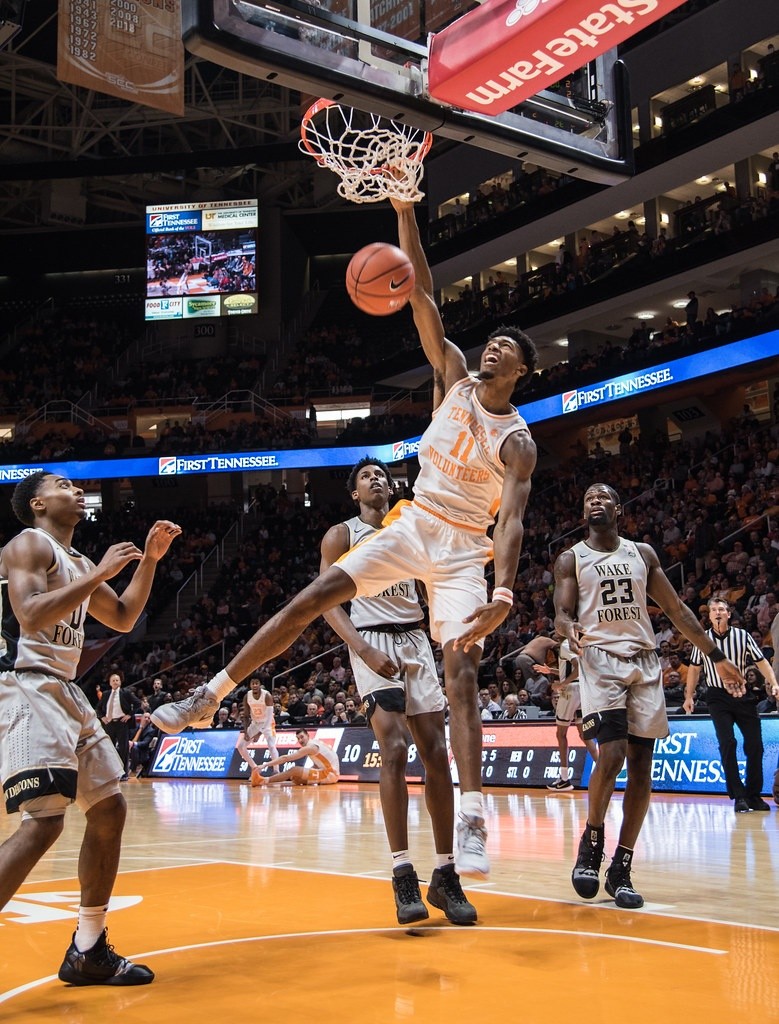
[503,684,509,686]
[489,687,496,689]
[668,674,679,678]
[515,672,521,675]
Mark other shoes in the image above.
[119,776,129,781]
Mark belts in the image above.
[110,719,123,722]
[518,652,534,660]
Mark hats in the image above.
[659,617,668,623]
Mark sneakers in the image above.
[770,783,779,804]
[425,863,477,925]
[572,830,605,900]
[604,858,644,908]
[453,812,491,881]
[149,683,221,735]
[734,795,750,813]
[546,775,575,791]
[750,795,770,810]
[251,769,262,787]
[391,864,429,925]
[57,925,156,986]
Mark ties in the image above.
[107,690,115,722]
[132,728,142,742]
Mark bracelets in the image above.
[492,587,514,606]
[708,647,727,662]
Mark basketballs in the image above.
[346,241,416,316]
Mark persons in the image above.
[554,483,747,908]
[151,168,536,879]
[730,63,745,102]
[684,291,698,324]
[443,151,779,325]
[318,456,477,923]
[683,598,779,813]
[146,235,256,296]
[237,675,279,780]
[252,728,340,786]
[0,312,779,771]
[0,470,183,985]
[96,673,143,782]
